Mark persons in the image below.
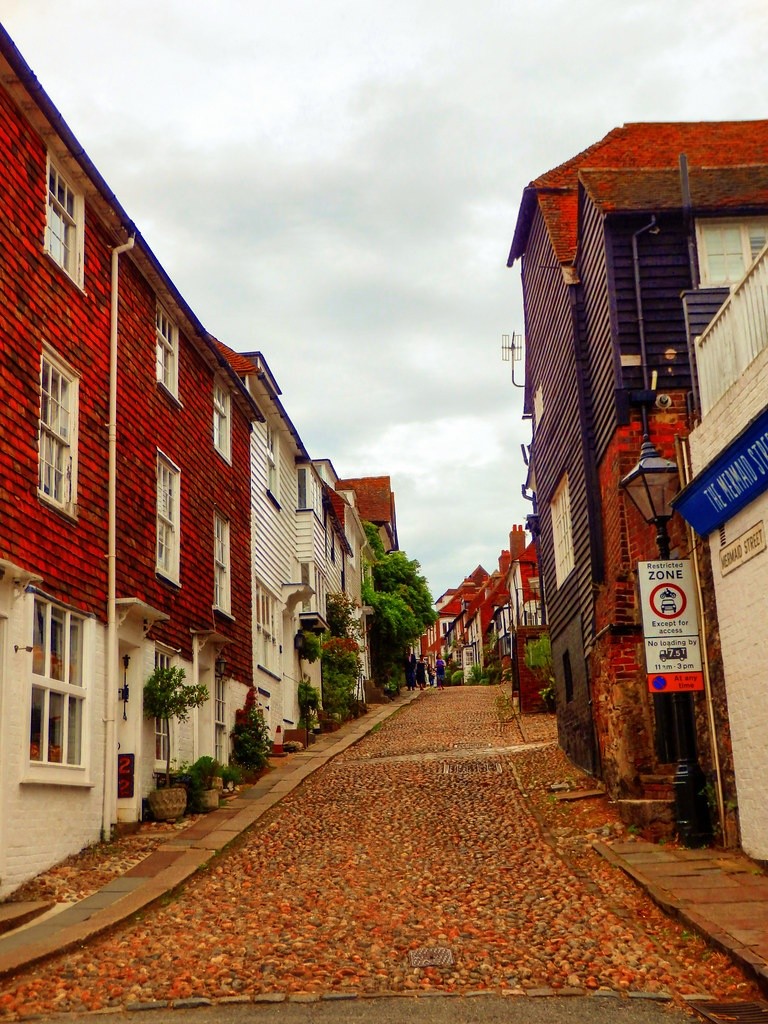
[435,653,446,691]
[416,654,427,691]
[427,664,435,686]
[402,647,417,691]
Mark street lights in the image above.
[619,405,714,850]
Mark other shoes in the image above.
[406,684,444,691]
